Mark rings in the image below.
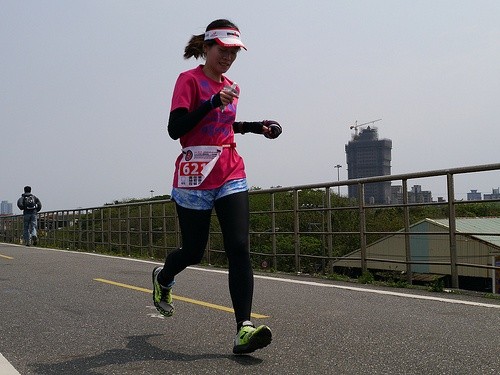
[229,96,231,100]
[228,90,231,94]
[227,100,230,104]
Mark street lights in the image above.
[149,190,155,198]
[333,164,344,196]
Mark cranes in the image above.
[349,118,384,139]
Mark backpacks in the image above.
[22,194,36,214]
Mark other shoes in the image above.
[32,236,37,245]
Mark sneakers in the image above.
[233,320,273,354]
[152,266,175,317]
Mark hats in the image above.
[204,28,247,51]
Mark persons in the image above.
[152,19,282,355]
[16,186,42,247]
